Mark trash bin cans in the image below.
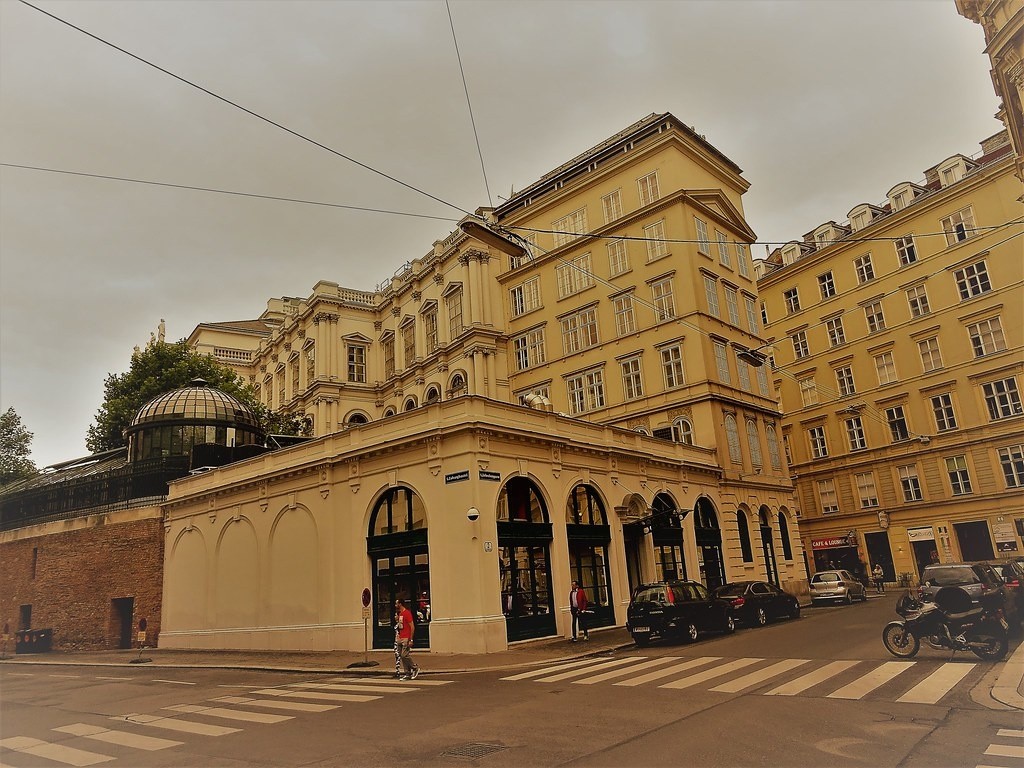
[16,628,35,654]
[32,627,51,653]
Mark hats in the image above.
[423,592,426,595]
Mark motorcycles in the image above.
[883,582,1009,662]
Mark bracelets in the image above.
[410,639,413,642]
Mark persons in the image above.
[419,591,429,620]
[504,585,512,617]
[872,564,885,594]
[393,598,421,682]
[570,581,590,641]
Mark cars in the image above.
[920,557,1023,626]
[808,569,866,604]
[626,583,801,644]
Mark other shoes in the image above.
[584,635,589,641]
[392,674,399,680]
[573,637,577,642]
[411,667,421,680]
[399,674,413,682]
